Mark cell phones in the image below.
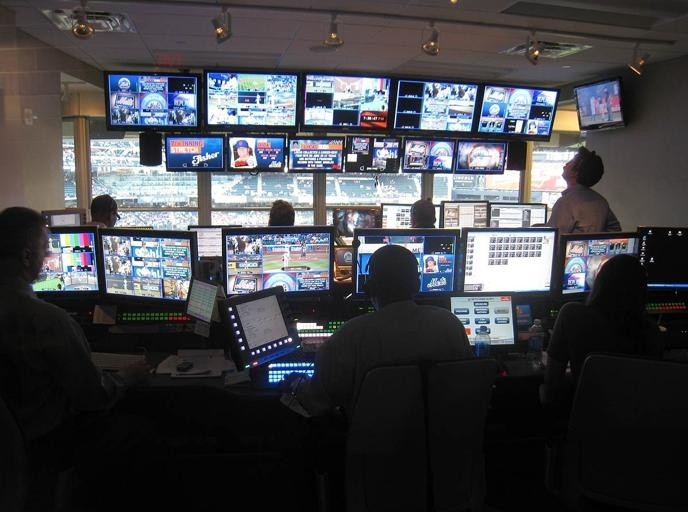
[177,360,194,372]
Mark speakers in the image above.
[510,140,531,171]
[138,130,172,166]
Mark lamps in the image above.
[322,13,345,47]
[526,30,545,64]
[420,23,440,56]
[210,7,231,43]
[628,43,647,76]
[71,0,96,40]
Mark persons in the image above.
[304,74,390,130]
[425,255,436,272]
[376,141,389,169]
[410,199,436,228]
[278,245,474,511]
[419,83,477,132]
[340,209,362,234]
[293,141,299,151]
[0,206,153,512]
[532,146,623,233]
[268,200,294,226]
[590,88,614,123]
[536,255,666,452]
[233,140,254,168]
[207,73,297,126]
[101,234,193,302]
[226,233,327,292]
[91,194,121,229]
[109,75,198,127]
[478,85,558,136]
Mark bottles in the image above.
[475,326,490,359]
[528,319,545,369]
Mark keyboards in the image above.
[89,350,144,374]
[257,358,317,385]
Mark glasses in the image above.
[114,213,121,221]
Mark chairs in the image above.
[544,352,688,511]
[0,398,78,512]
[315,358,498,511]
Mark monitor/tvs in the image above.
[103,68,201,133]
[203,64,300,134]
[164,133,224,173]
[221,224,336,300]
[225,135,287,173]
[573,78,630,133]
[187,222,239,259]
[97,225,195,308]
[395,73,478,137]
[449,293,525,352]
[440,199,488,229]
[638,222,687,288]
[462,225,558,298]
[331,203,382,232]
[220,286,303,370]
[380,201,442,229]
[353,224,461,306]
[455,139,509,175]
[402,138,452,174]
[346,135,400,173]
[303,69,391,132]
[480,81,560,142]
[29,224,102,304]
[559,231,640,301]
[289,137,344,171]
[490,200,548,230]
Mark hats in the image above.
[235,140,249,149]
[426,256,434,261]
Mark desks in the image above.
[117,348,688,400]
